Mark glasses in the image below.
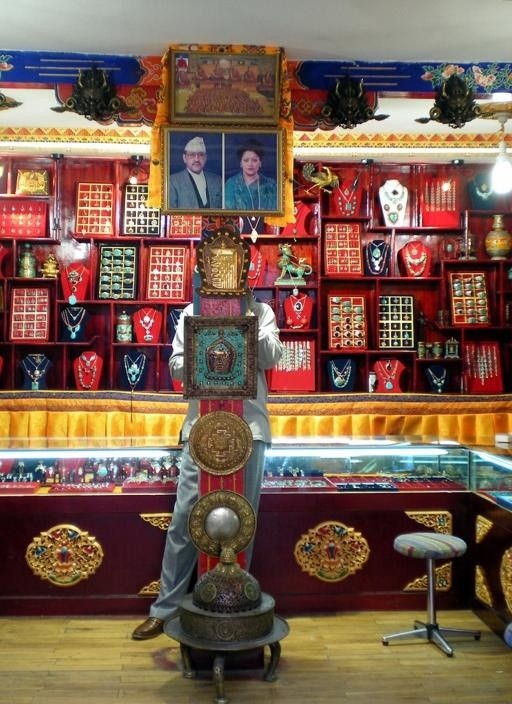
[185,151,206,160]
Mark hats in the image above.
[184,137,205,154]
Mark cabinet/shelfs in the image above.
[0,154,510,397]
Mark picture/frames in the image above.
[158,47,287,219]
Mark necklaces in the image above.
[22,355,50,390]
[376,358,399,389]
[241,172,261,209]
[425,367,448,394]
[330,168,363,217]
[288,202,304,243]
[61,306,86,339]
[124,353,147,390]
[63,260,88,305]
[404,240,426,275]
[329,357,352,389]
[285,261,306,296]
[246,250,262,302]
[365,241,390,275]
[78,353,98,389]
[138,308,157,342]
[381,184,406,222]
[284,294,309,329]
[470,176,496,198]
[169,311,180,331]
[246,216,261,243]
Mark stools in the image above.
[380,532,484,657]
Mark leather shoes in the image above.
[131,616,164,639]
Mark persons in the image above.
[169,136,222,208]
[131,231,282,640]
[224,139,277,209]
